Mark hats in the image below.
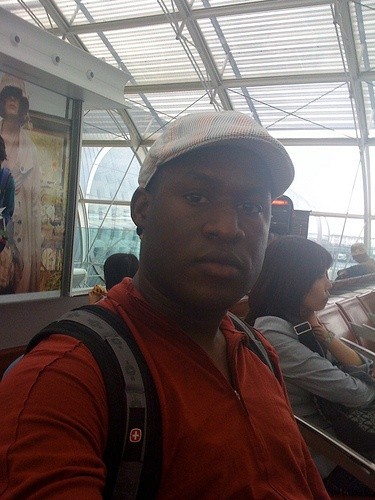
[0,75,30,123]
[138,109,295,206]
[350,242,367,256]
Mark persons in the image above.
[1,76,45,293]
[1,108,336,499]
[348,240,375,267]
[85,249,140,305]
[245,235,375,499]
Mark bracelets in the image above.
[323,331,336,345]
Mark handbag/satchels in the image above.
[0,242,15,289]
[267,306,373,452]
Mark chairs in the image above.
[226,272,375,500]
